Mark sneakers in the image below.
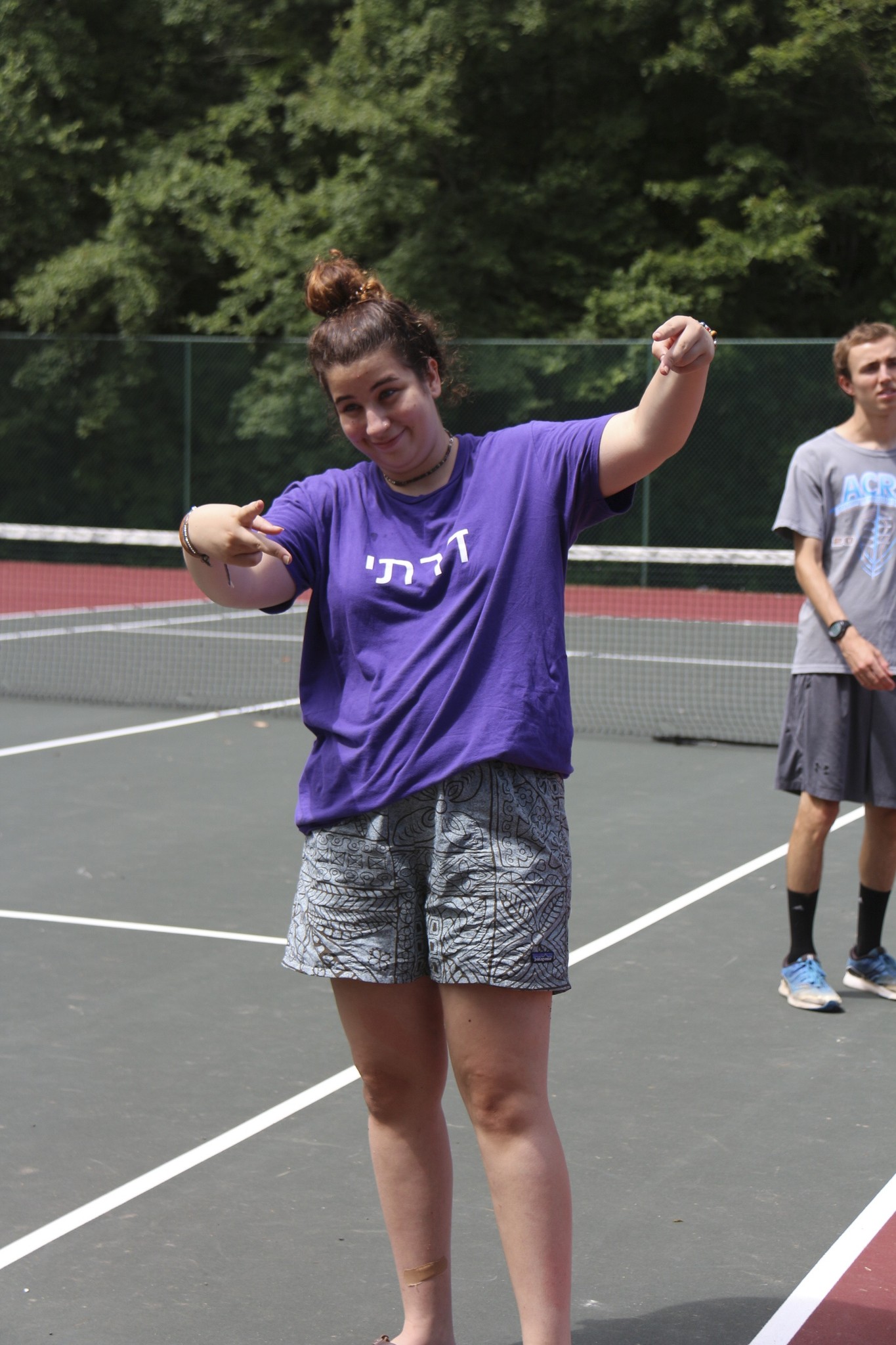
[777,951,843,1010]
[845,946,896,1000]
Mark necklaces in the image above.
[382,428,453,487]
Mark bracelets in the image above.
[179,506,236,588]
[699,319,718,349]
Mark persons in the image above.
[179,248,715,1345]
[772,320,896,1011]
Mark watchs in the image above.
[827,620,852,642]
